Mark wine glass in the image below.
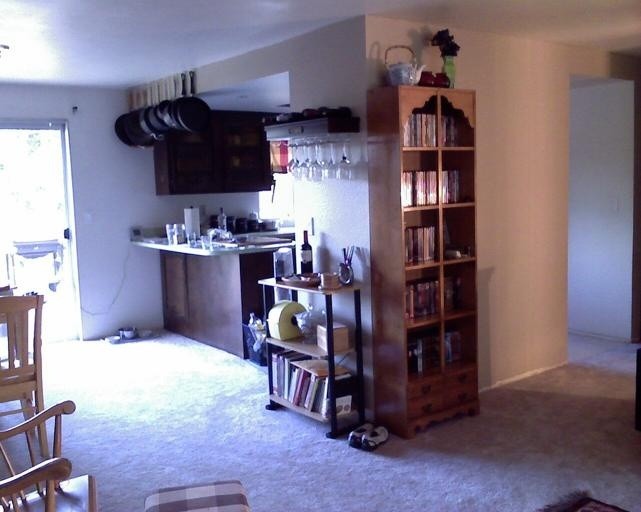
[287,137,352,182]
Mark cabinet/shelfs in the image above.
[367,84,478,441]
[258,276,364,439]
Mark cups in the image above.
[166,223,185,246]
[321,271,339,288]
[187,232,211,251]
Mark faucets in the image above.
[208,229,233,251]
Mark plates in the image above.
[281,272,322,288]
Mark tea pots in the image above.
[384,44,428,87]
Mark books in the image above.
[402,113,461,377]
[268,350,358,419]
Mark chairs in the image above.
[0,293,95,512]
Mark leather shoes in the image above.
[348,423,390,452]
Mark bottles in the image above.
[248,312,264,342]
[301,230,314,274]
[217,207,228,232]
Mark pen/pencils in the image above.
[342,246,354,265]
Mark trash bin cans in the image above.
[242,320,270,366]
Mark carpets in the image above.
[538,488,628,512]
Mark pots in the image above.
[114,71,211,149]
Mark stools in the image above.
[144,480,251,512]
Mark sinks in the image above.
[214,234,292,248]
